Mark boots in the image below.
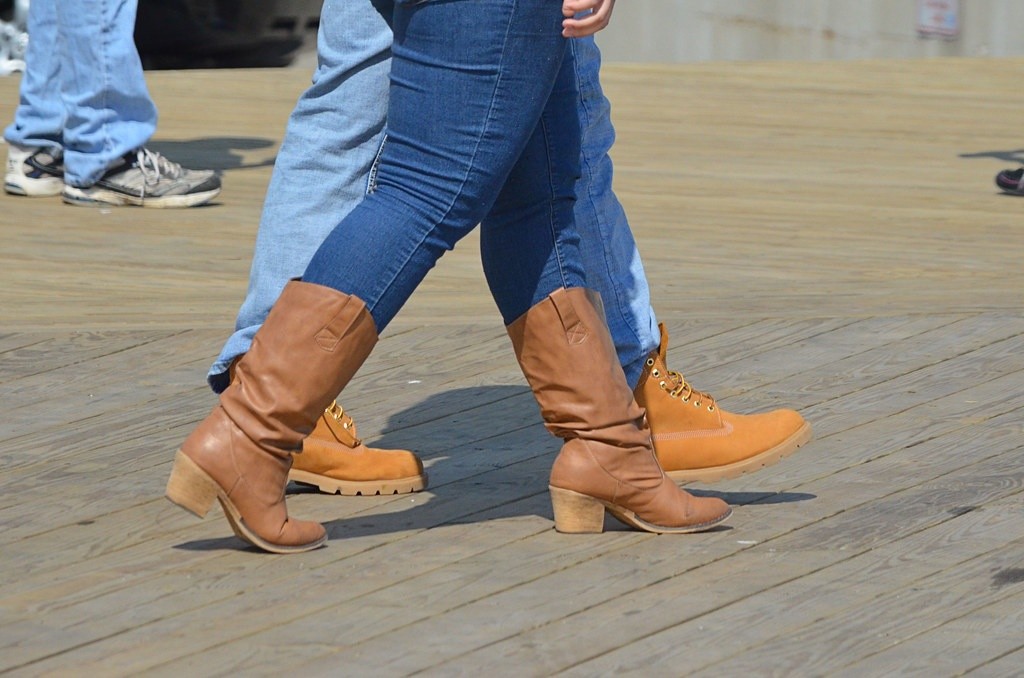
[505,286,734,533]
[229,354,429,496]
[164,276,379,554]
[633,322,812,483]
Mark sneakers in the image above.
[4,142,221,208]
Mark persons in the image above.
[0,1,222,213]
[206,0,813,495]
[165,0,728,555]
[995,166,1024,196]
